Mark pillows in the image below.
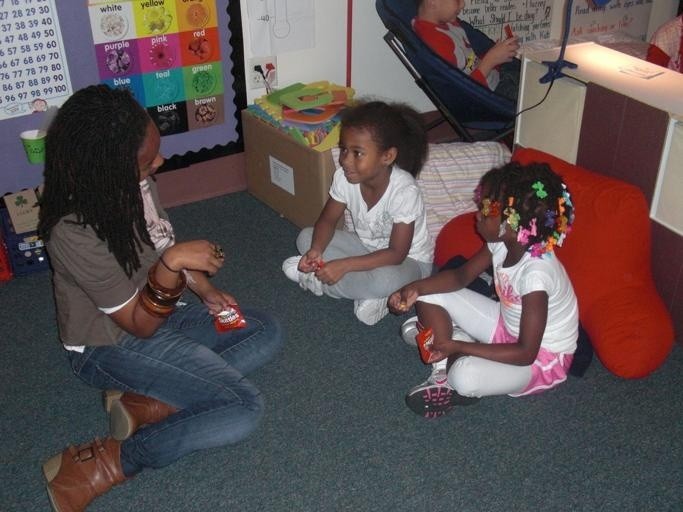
[430,143,675,379]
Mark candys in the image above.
[400,304,406,309]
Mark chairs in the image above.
[373,0,513,147]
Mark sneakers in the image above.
[405,367,482,419]
[282,255,323,298]
[400,315,477,347]
[353,297,390,327]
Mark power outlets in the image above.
[248,55,278,90]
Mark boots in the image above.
[42,436,135,512]
[103,389,178,442]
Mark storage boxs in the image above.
[235,106,344,230]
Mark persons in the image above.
[386,160,580,419]
[31,82,287,512]
[283,101,437,326]
[646,0,683,75]
[411,0,521,102]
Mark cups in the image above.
[18,129,46,165]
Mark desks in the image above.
[513,40,683,237]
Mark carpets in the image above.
[0,183,682,510]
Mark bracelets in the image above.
[138,267,187,319]
[159,256,181,273]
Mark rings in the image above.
[215,247,224,259]
